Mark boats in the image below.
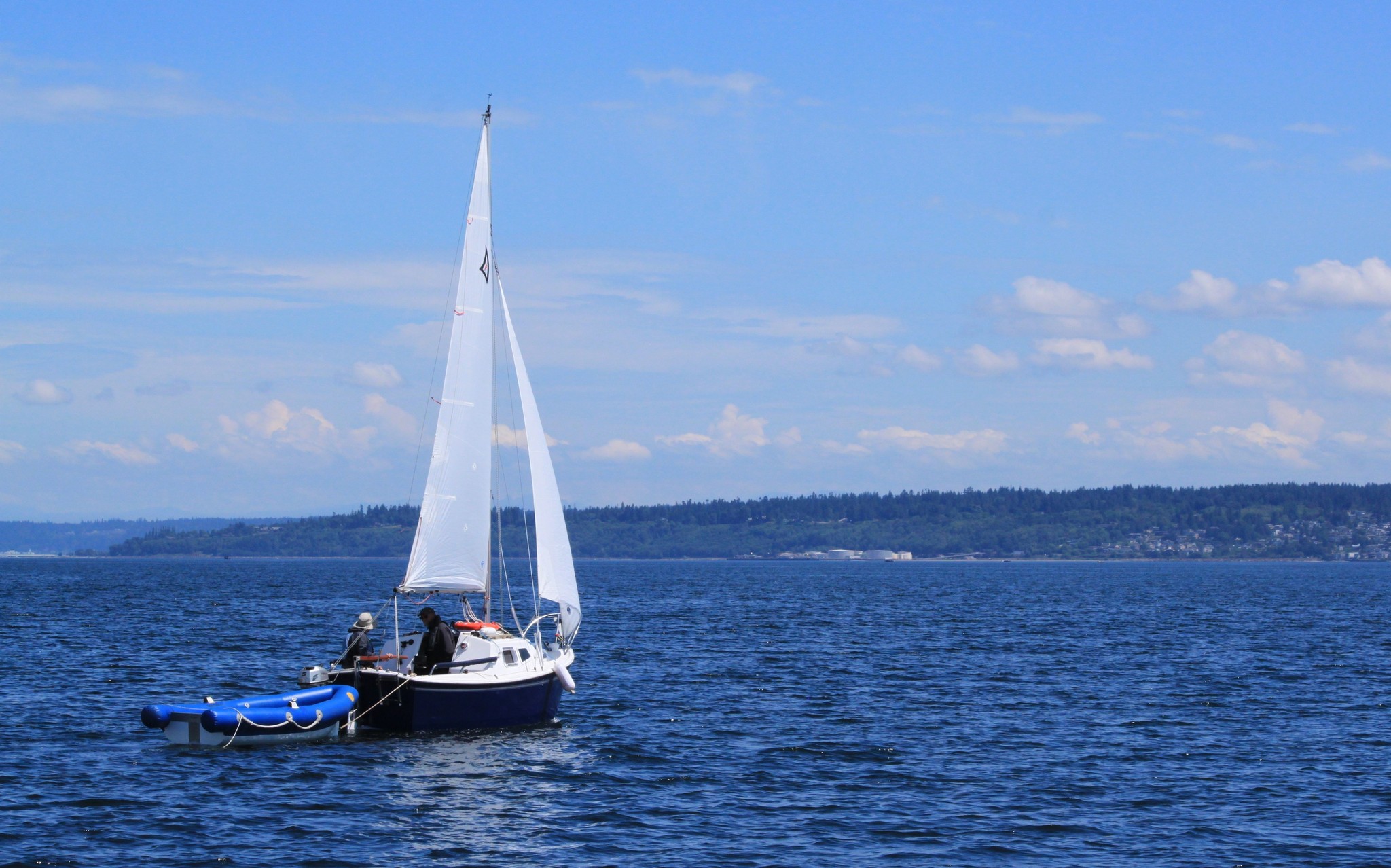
[140,685,360,747]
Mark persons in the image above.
[341,612,393,669]
[417,607,457,674]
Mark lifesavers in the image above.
[453,621,501,631]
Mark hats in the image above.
[417,607,435,617]
[353,612,379,630]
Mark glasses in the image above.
[419,615,428,620]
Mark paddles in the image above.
[353,652,408,662]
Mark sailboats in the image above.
[298,94,582,737]
